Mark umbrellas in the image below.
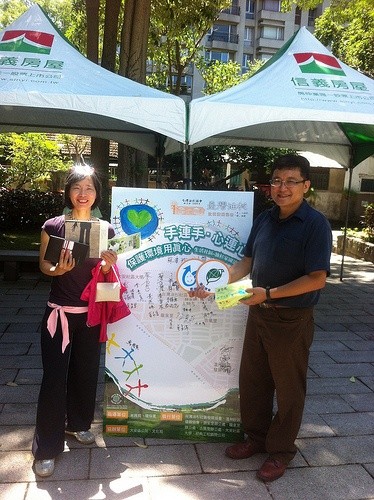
[1,0,190,220]
[189,26,373,288]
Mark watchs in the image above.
[265,286,272,303]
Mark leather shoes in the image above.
[257,457,291,481]
[225,442,254,458]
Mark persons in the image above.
[43,234,90,267]
[27,164,119,478]
[192,153,334,483]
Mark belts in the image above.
[258,302,289,308]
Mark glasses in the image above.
[268,179,305,187]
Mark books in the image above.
[63,220,108,259]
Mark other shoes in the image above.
[65,430,94,444]
[34,458,54,477]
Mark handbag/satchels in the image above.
[95,265,122,303]
